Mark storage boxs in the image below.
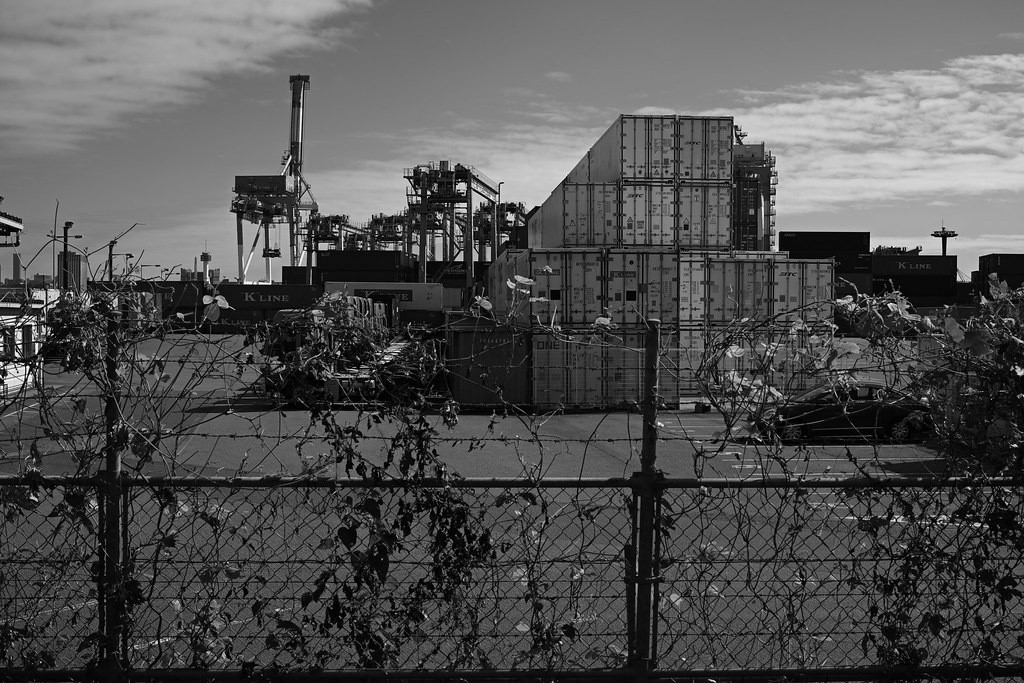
[323,281,443,312]
[443,115,836,411]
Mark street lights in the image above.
[930,227,958,256]
[55,223,85,294]
[141,263,181,281]
[108,239,134,283]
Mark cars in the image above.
[747,380,935,444]
